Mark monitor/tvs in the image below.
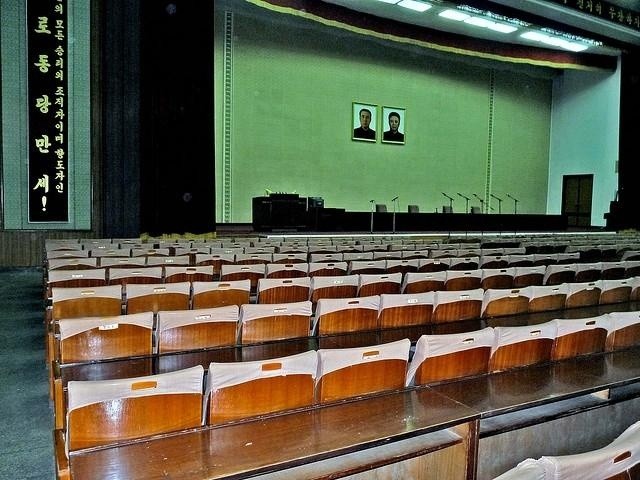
[308,197,324,208]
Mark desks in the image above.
[68,383,481,480]
[426,344,640,480]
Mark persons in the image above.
[383,112,404,142]
[354,109,375,139]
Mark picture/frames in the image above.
[381,105,406,145]
[352,102,378,143]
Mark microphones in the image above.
[391,196,398,201]
[369,200,375,202]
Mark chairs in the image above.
[408,205,419,212]
[203,349,318,426]
[471,207,480,213]
[376,204,387,212]
[606,311,640,350]
[489,319,558,371]
[494,459,545,480]
[317,337,411,406]
[553,313,610,361]
[443,205,453,212]
[53,364,204,480]
[540,420,640,480]
[406,327,494,386]
